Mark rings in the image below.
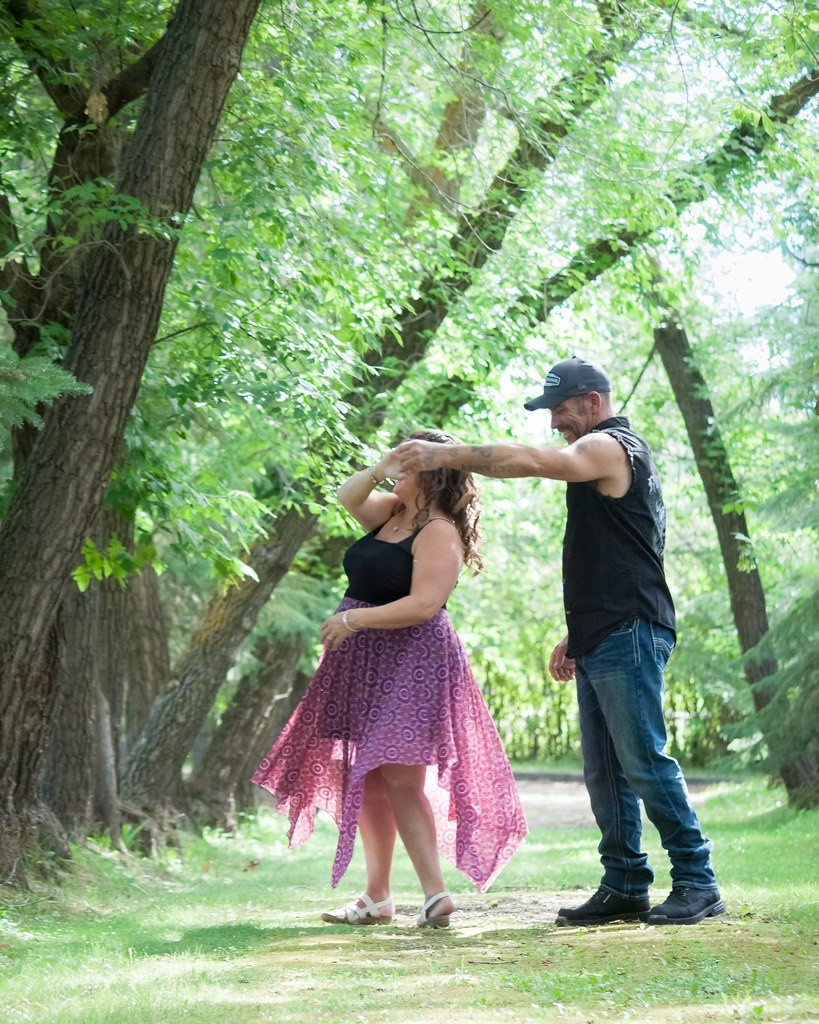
[326,636,332,642]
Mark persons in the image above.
[397,355,727,927]
[248,428,529,927]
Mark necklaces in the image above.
[393,512,413,532]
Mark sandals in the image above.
[417,891,451,928]
[322,893,394,925]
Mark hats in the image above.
[524,356,611,411]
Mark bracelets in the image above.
[368,464,385,486]
[342,608,361,633]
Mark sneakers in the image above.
[648,886,726,925]
[558,888,650,925]
